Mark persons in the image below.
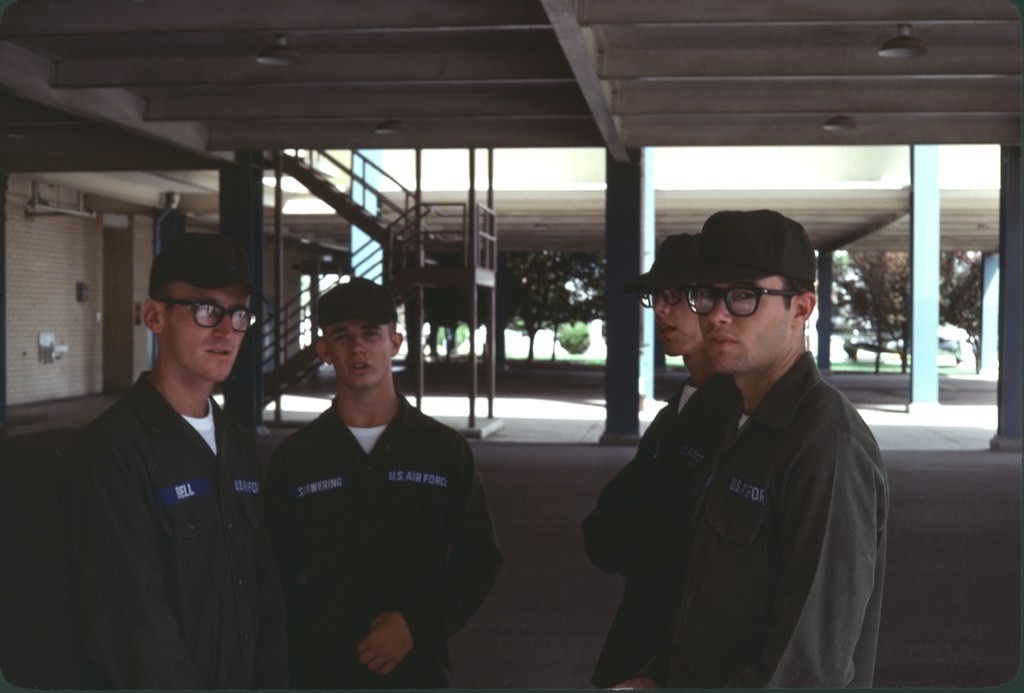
[607,209,890,693]
[68,232,287,689]
[264,278,504,693]
[581,235,744,689]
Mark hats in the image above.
[698,211,817,285]
[315,281,397,322]
[147,231,266,301]
[623,232,704,293]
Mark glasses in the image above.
[156,300,255,334]
[687,283,805,317]
[638,288,686,309]
